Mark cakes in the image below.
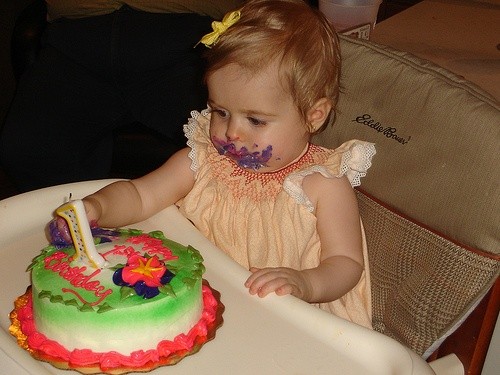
[7,227,225,375]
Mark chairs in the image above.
[307,32,500,375]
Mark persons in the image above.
[50,0,376,330]
[0,0,245,193]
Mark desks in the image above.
[0,179,436,375]
